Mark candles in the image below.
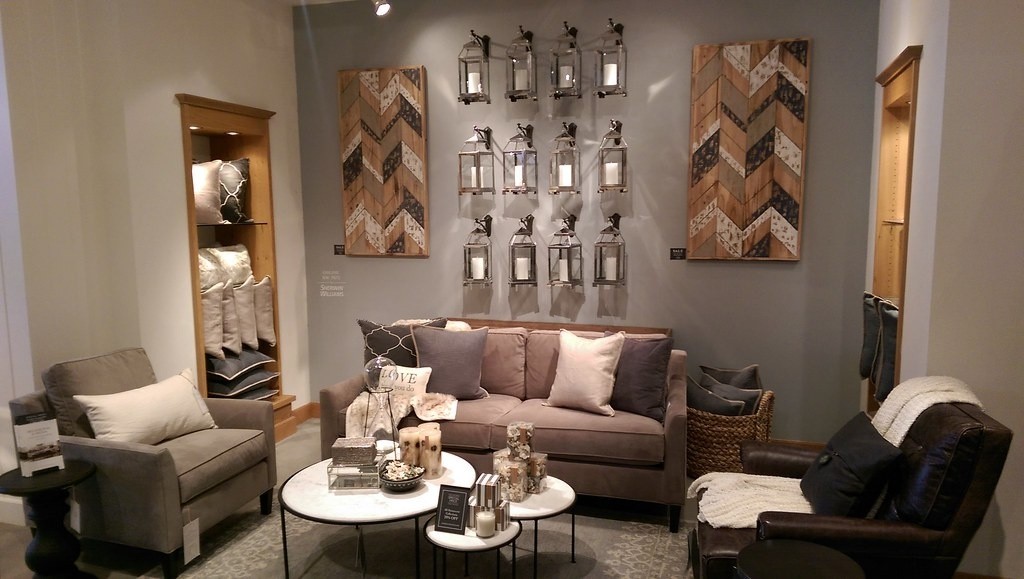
[471,256,484,280]
[515,256,528,281]
[475,512,496,538]
[604,161,619,185]
[471,166,483,187]
[558,258,570,283]
[514,164,525,186]
[513,69,529,91]
[559,66,573,89]
[558,165,571,187]
[397,419,442,480]
[466,72,480,93]
[605,257,616,279]
[603,63,617,86]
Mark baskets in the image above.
[686,387,777,477]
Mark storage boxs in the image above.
[326,453,386,489]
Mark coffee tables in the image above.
[424,522,522,579]
[472,474,576,579]
[281,448,476,578]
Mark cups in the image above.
[475,511,496,538]
[399,422,442,480]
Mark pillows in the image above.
[604,331,673,424]
[800,410,902,524]
[192,160,230,225]
[858,290,898,401]
[236,273,259,353]
[353,317,446,379]
[201,283,225,360]
[409,324,490,401]
[379,366,433,398]
[542,328,626,418]
[220,158,254,223]
[687,362,762,415]
[200,243,254,288]
[205,348,276,381]
[255,274,277,349]
[207,371,281,397]
[222,277,243,357]
[74,368,217,446]
[241,388,280,403]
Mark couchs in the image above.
[10,347,276,579]
[319,317,688,534]
[684,394,1013,579]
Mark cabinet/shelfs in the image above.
[853,45,921,409]
[175,93,283,413]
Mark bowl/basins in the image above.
[379,464,425,490]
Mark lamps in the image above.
[370,1,392,16]
